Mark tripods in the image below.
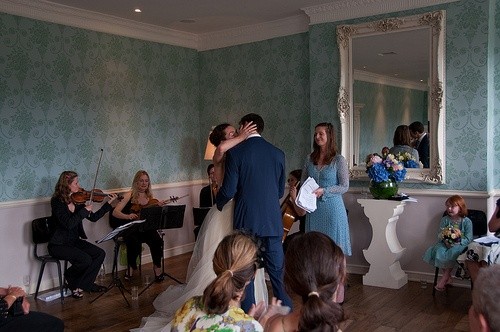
[88,224,134,307]
[137,203,187,294]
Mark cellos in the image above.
[280,178,300,244]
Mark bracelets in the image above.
[6,295,16,301]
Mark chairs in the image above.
[107,210,166,275]
[431,208,488,299]
[31,216,73,301]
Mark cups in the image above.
[420,279,427,288]
[361,191,367,199]
[131,286,138,300]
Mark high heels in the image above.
[65,283,84,298]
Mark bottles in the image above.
[100,264,105,281]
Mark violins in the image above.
[71,187,124,206]
[129,196,179,220]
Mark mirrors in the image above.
[335,10,447,185]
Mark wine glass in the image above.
[24,275,32,297]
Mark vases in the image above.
[370,180,398,199]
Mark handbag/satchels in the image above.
[9,297,24,317]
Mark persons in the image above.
[389,125,419,164]
[382,147,390,157]
[469,265,500,332]
[130,123,268,332]
[290,122,349,306]
[457,199,500,285]
[409,121,430,168]
[423,195,473,292]
[171,234,281,332]
[284,169,305,231]
[214,113,293,316]
[48,171,118,299]
[0,285,64,332]
[263,231,348,332]
[113,171,171,282]
[194,164,220,242]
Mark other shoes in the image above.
[124,267,134,281]
[435,279,454,292]
[153,265,165,282]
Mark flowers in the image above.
[366,152,406,184]
[396,151,423,169]
[437,224,463,249]
